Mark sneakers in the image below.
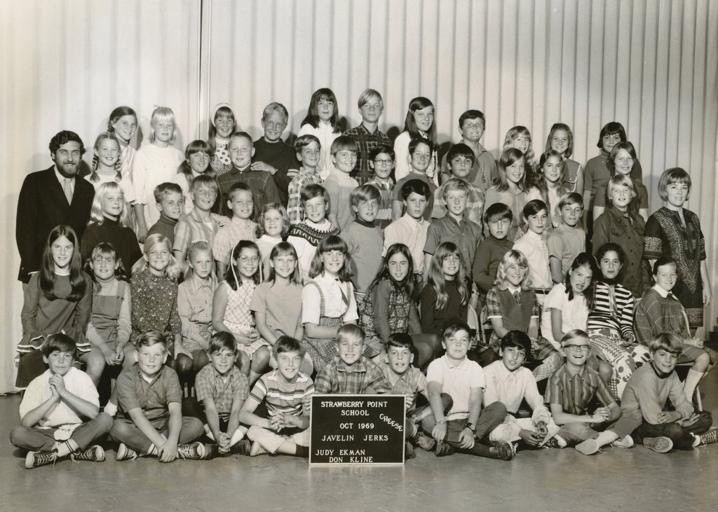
[574,427,716,455]
[404,433,514,461]
[24,442,104,469]
[117,440,251,462]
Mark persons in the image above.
[10,88,717,469]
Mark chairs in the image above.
[633,297,704,412]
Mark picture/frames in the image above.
[309,393,406,465]
